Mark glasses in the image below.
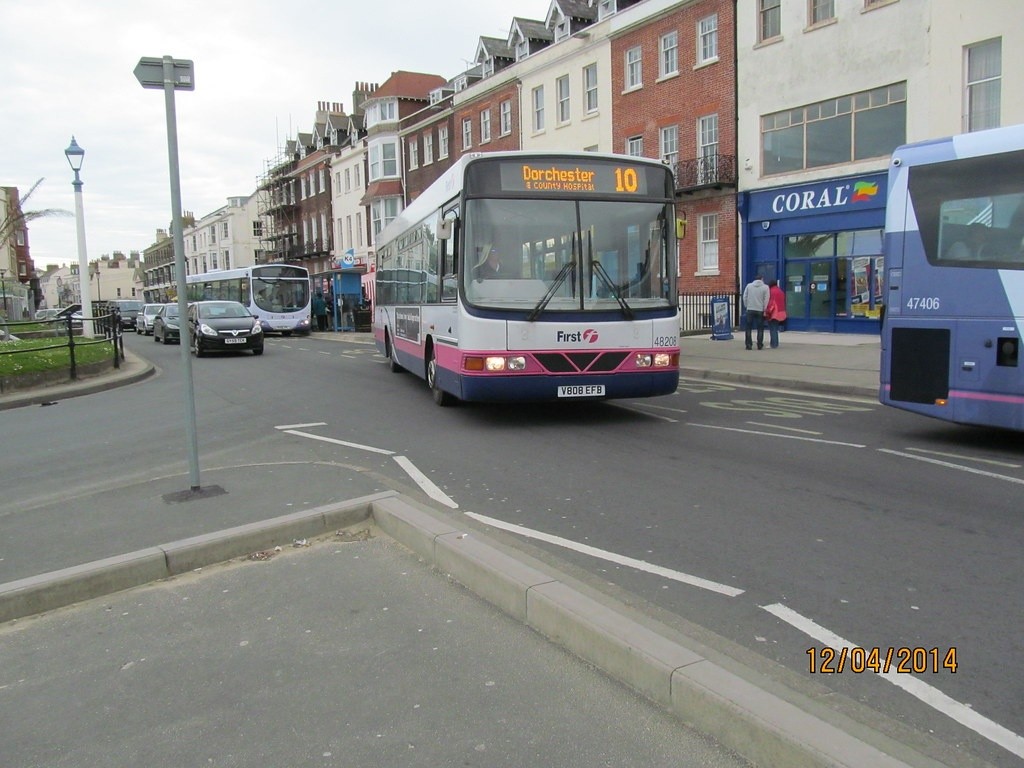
[491,248,496,254]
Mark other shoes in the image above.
[758,344,763,350]
[315,329,320,332]
[770,342,778,349]
[746,344,752,349]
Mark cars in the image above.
[188,301,264,357]
[153,303,192,344]
[136,303,166,336]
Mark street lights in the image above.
[95,269,101,316]
[65,135,95,340]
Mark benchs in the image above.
[941,222,1005,262]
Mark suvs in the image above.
[35,308,83,322]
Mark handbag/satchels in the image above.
[766,300,776,318]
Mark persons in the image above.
[479,247,504,279]
[742,274,787,351]
[314,292,349,332]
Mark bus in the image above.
[374,150,687,406]
[878,123,1024,433]
[186,264,312,337]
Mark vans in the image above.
[105,300,144,332]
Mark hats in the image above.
[768,279,776,287]
